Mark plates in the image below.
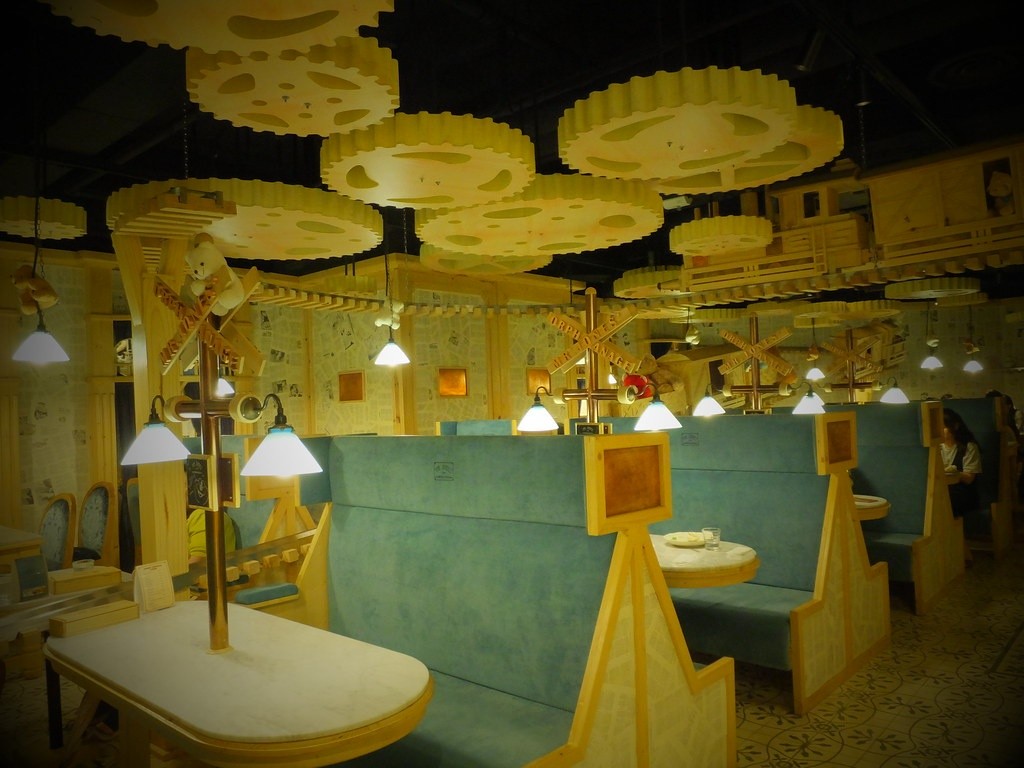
[663,532,713,547]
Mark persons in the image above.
[944,407,984,513]
[985,390,1024,463]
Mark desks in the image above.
[649,533,760,590]
[42,599,435,768]
[854,493,892,522]
[0,524,45,606]
[945,471,962,486]
[1008,441,1021,457]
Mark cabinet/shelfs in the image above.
[858,135,1024,269]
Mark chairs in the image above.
[14,491,77,592]
[77,478,121,568]
[126,478,143,571]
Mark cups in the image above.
[702,527,720,551]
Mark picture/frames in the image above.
[338,369,366,403]
[525,366,552,397]
[435,366,469,399]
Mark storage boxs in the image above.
[48,598,139,639]
[48,565,122,594]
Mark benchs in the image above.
[209,396,1024,768]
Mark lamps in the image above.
[690,382,754,420]
[803,318,825,382]
[372,210,411,370]
[596,383,683,432]
[961,305,984,374]
[919,299,944,371]
[120,393,202,466]
[10,128,70,366]
[853,376,910,404]
[515,385,588,432]
[215,377,235,398]
[758,381,825,415]
[206,394,324,477]
[805,381,850,407]
